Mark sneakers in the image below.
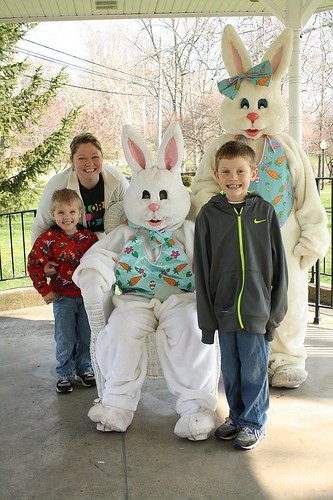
[233,426,266,449]
[55,377,73,392]
[74,370,96,386]
[215,417,242,440]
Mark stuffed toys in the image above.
[191,24,331,388]
[87,122,222,438]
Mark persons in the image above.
[27,190,97,393]
[193,141,289,450]
[31,133,131,239]
